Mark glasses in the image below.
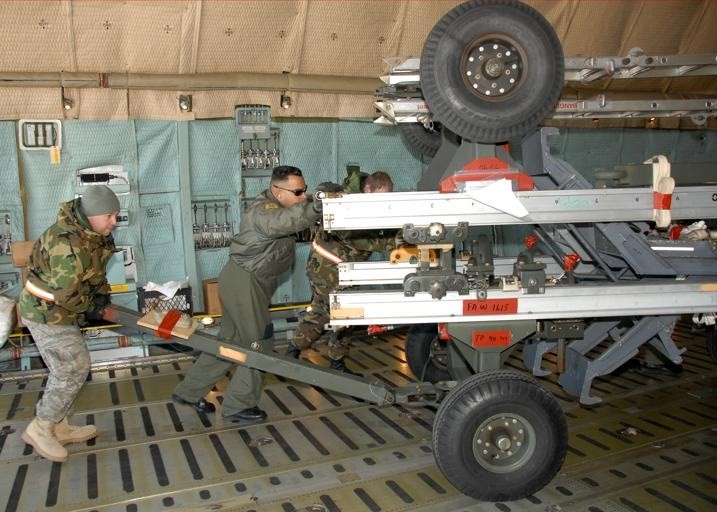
[271,183,307,197]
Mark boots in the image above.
[328,357,364,379]
[18,414,70,463]
[283,343,301,360]
[52,413,99,447]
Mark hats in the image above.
[76,183,122,220]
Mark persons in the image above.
[170,164,350,425]
[11,184,122,464]
[284,170,409,378]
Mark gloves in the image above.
[311,180,346,214]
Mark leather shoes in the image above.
[171,388,216,414]
[221,405,267,423]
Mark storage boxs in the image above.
[202,278,224,317]
